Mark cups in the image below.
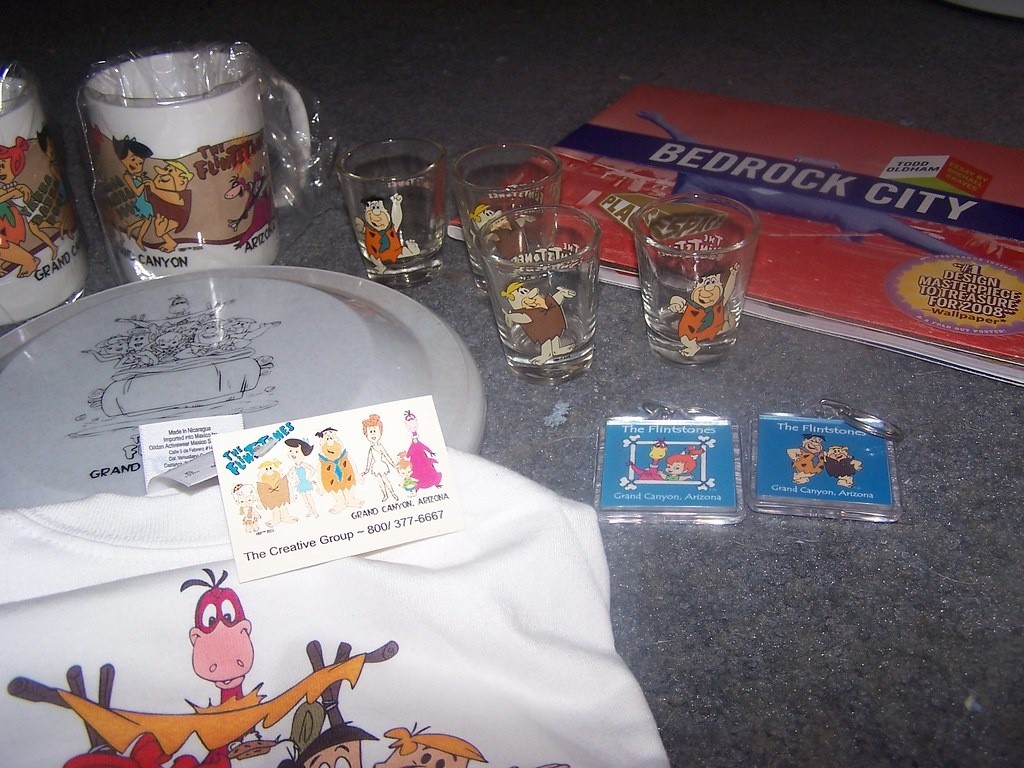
[81,49,311,283]
[451,141,562,292]
[475,205,602,384]
[635,193,758,365]
[339,135,447,290]
[0,74,86,327]
